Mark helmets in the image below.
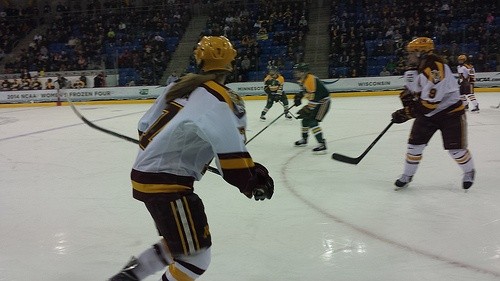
[292,62,313,82]
[457,54,467,64]
[267,64,278,75]
[195,34,238,73]
[406,36,435,58]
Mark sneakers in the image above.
[260,114,266,121]
[284,113,292,119]
[293,135,309,147]
[462,169,474,193]
[463,102,469,111]
[470,103,480,112]
[312,139,329,154]
[393,172,413,191]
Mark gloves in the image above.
[246,162,274,201]
[296,105,311,118]
[293,92,303,106]
[269,92,281,103]
[389,89,414,123]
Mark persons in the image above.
[392,37,476,190]
[293,63,330,155]
[468,63,475,95]
[260,66,292,120]
[109,35,274,281]
[457,55,478,112]
[0,0,500,91]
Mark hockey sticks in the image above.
[331,121,394,165]
[66,98,266,200]
[278,101,302,120]
[245,104,295,145]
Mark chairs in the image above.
[11,14,500,88]
